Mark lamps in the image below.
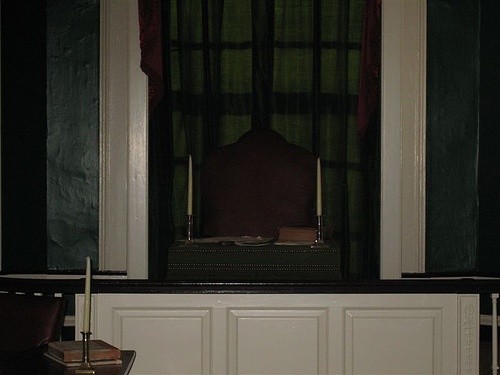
[76,256,96,375]
[309,156,329,249]
[180,154,198,248]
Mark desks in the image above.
[1,349,137,375]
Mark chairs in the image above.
[1,303,65,375]
